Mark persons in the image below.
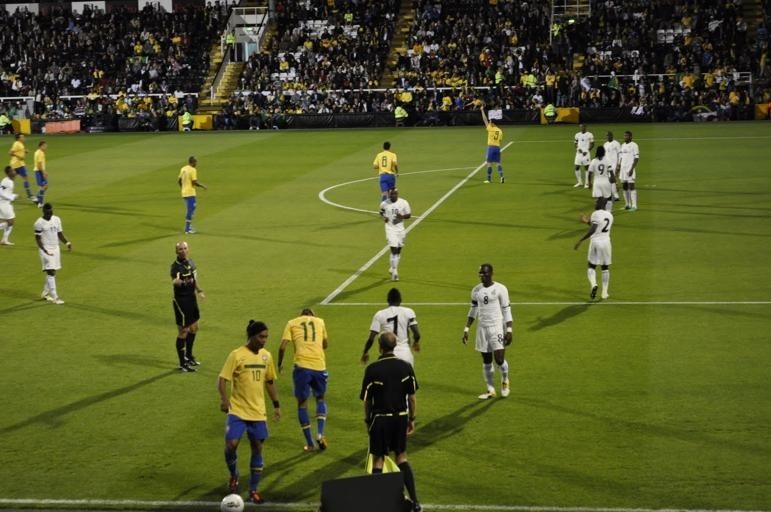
[1,0,771,132]
[379,188,412,281]
[0,165,19,245]
[218,288,421,512]
[170,241,206,372]
[373,141,399,202]
[33,141,47,208]
[480,106,505,183]
[574,123,639,299]
[8,134,34,199]
[33,204,71,304]
[463,264,513,399]
[178,156,207,234]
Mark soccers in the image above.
[220,493,245,510]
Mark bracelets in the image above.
[65,241,70,246]
[197,289,203,294]
[463,327,469,332]
[506,327,513,333]
[34,233,42,236]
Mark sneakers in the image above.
[41,290,54,301]
[185,230,197,235]
[483,180,494,183]
[0,240,15,246]
[628,206,639,211]
[248,489,262,503]
[601,292,610,300]
[478,391,497,399]
[619,204,632,210]
[584,183,591,189]
[317,436,327,450]
[184,355,200,366]
[574,182,584,188]
[412,501,422,512]
[391,270,398,282]
[500,379,511,398]
[178,362,199,372]
[52,297,65,305]
[590,284,598,299]
[499,176,504,184]
[304,445,316,453]
[228,472,240,493]
